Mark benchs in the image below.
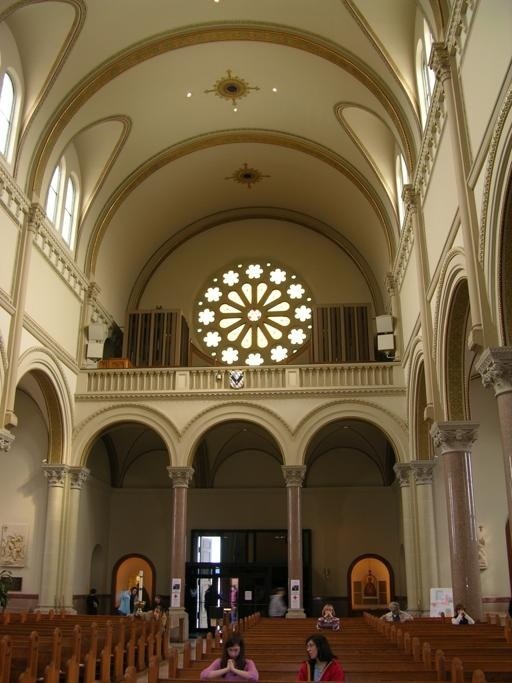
[149,612,510,680]
[1,608,172,682]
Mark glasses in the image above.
[305,643,316,647]
[324,608,333,610]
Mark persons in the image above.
[198,634,261,681]
[202,583,230,629]
[450,603,476,626]
[150,594,169,612]
[295,633,345,682]
[316,603,342,631]
[380,599,416,624]
[115,586,139,616]
[266,586,288,618]
[134,581,151,609]
[85,587,100,614]
[229,583,238,622]
[133,605,169,633]
